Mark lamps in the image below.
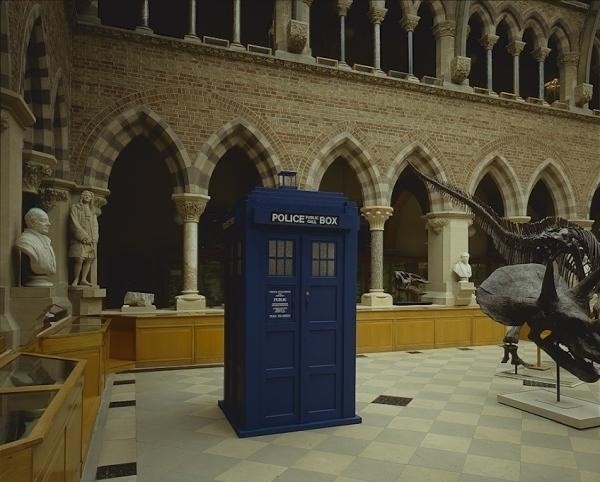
[43,304,68,326]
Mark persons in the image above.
[67,190,96,286]
[9,207,58,275]
